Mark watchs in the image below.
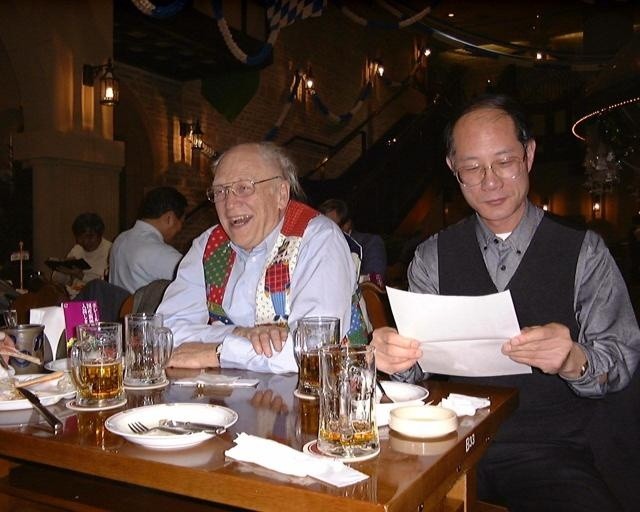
[216,343,223,364]
[581,361,588,376]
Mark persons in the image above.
[55,212,113,293]
[365,93,640,512]
[0,331,16,366]
[316,198,388,279]
[108,186,188,296]
[151,141,373,375]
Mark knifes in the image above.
[16,386,64,429]
[162,417,225,434]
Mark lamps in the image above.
[418,47,429,64]
[180,119,205,150]
[366,57,385,82]
[82,59,120,106]
[297,63,317,91]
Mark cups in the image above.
[294,316,339,395]
[317,345,382,457]
[7,321,46,373]
[65,319,125,405]
[78,410,124,450]
[124,312,174,385]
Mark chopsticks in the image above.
[196,368,205,388]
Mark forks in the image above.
[128,419,190,436]
[374,374,395,402]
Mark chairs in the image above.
[79,281,131,322]
[11,291,67,326]
[121,280,171,316]
[359,282,396,330]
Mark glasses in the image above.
[207,177,281,204]
[448,146,527,190]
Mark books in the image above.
[44,257,91,271]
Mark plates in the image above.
[0,374,74,411]
[42,356,74,372]
[104,401,239,450]
[369,379,428,423]
